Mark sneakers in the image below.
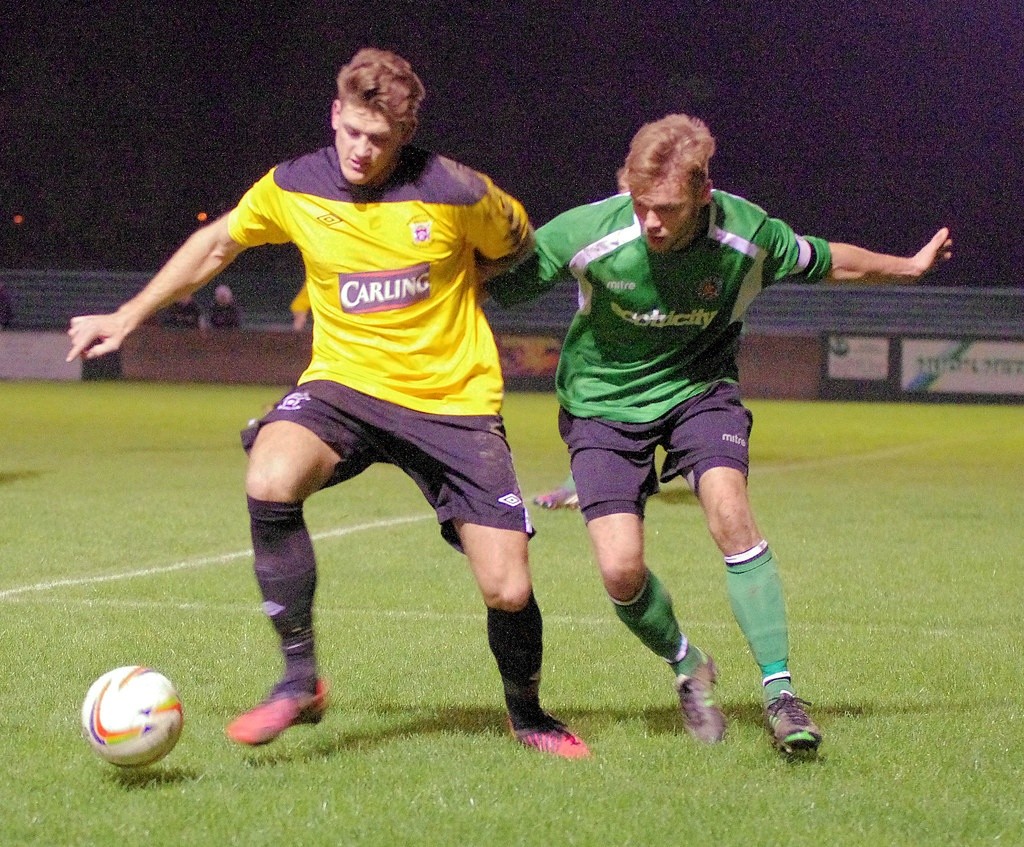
[507,712,591,759]
[759,679,822,754]
[677,646,725,747]
[226,676,330,746]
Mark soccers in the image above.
[77,666,184,766]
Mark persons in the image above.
[66,47,590,761]
[160,294,206,329]
[473,112,952,752]
[290,279,311,329]
[208,286,241,329]
[530,166,633,508]
